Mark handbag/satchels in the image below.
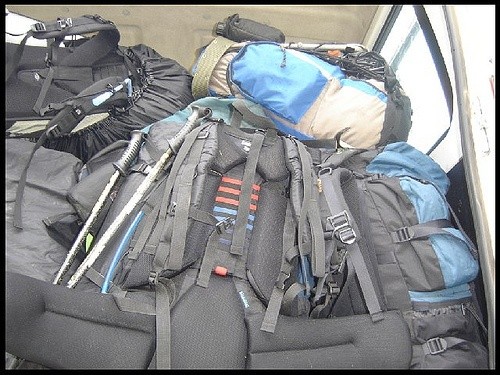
[215,14,285,44]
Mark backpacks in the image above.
[65,96,490,370]
[6,15,195,164]
[191,35,413,149]
